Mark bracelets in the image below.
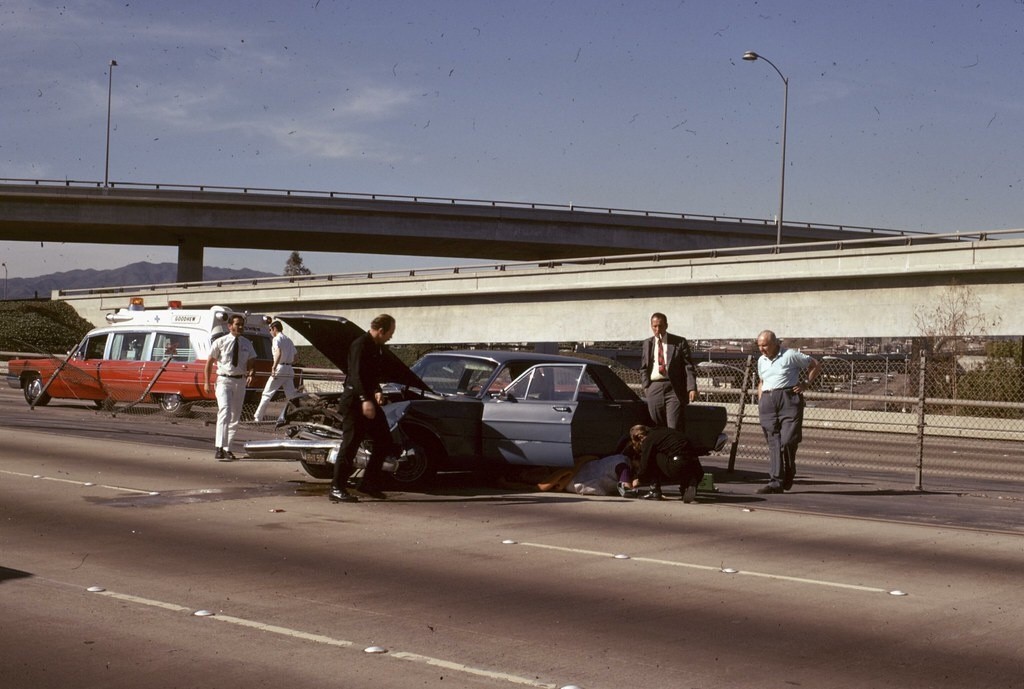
[248,375,253,377]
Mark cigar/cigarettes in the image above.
[660,333,662,339]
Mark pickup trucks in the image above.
[5,324,303,418]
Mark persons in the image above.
[630,425,704,503]
[755,330,821,495]
[204,314,258,459]
[640,313,697,433]
[565,448,641,496]
[253,321,298,425]
[329,314,396,502]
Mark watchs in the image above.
[804,380,808,383]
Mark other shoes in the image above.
[683,486,697,503]
[643,489,661,500]
[756,486,783,494]
[215,451,224,458]
[223,450,235,459]
[784,476,793,490]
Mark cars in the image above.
[887,375,894,382]
[871,377,882,385]
[834,385,842,393]
[272,311,731,493]
[836,346,857,352]
[851,373,866,387]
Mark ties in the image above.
[658,337,666,376]
[232,337,239,368]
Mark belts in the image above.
[221,374,242,378]
[650,379,671,382]
[281,363,291,365]
[763,386,794,392]
[345,385,354,390]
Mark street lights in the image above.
[104,59,119,188]
[891,350,908,413]
[742,48,790,254]
[866,353,889,413]
[823,356,853,410]
[697,362,756,404]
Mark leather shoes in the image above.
[356,477,387,499]
[328,489,358,503]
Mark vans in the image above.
[104,298,272,366]
